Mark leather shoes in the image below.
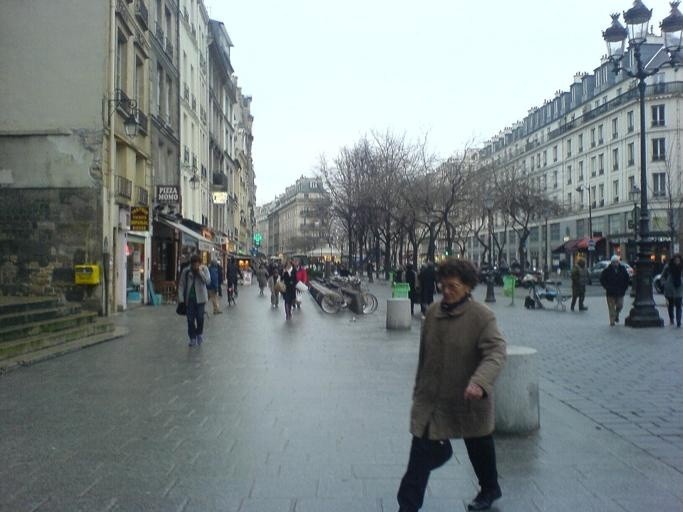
[189,339,196,346]
[467,487,501,512]
[213,311,223,314]
[196,335,202,345]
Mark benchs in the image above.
[525,281,572,313]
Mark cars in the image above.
[472,261,543,288]
[580,256,636,288]
[650,264,671,295]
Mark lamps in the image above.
[102,88,137,139]
[178,164,201,189]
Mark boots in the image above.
[289,308,292,319]
[284,304,290,320]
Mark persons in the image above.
[395,259,506,512]
[475,250,683,329]
[171,247,441,345]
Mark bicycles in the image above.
[305,272,380,318]
[227,274,244,307]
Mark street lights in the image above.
[479,194,499,305]
[596,0,682,331]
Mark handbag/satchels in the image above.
[177,303,186,315]
[295,289,302,305]
[430,437,453,471]
[273,275,288,293]
